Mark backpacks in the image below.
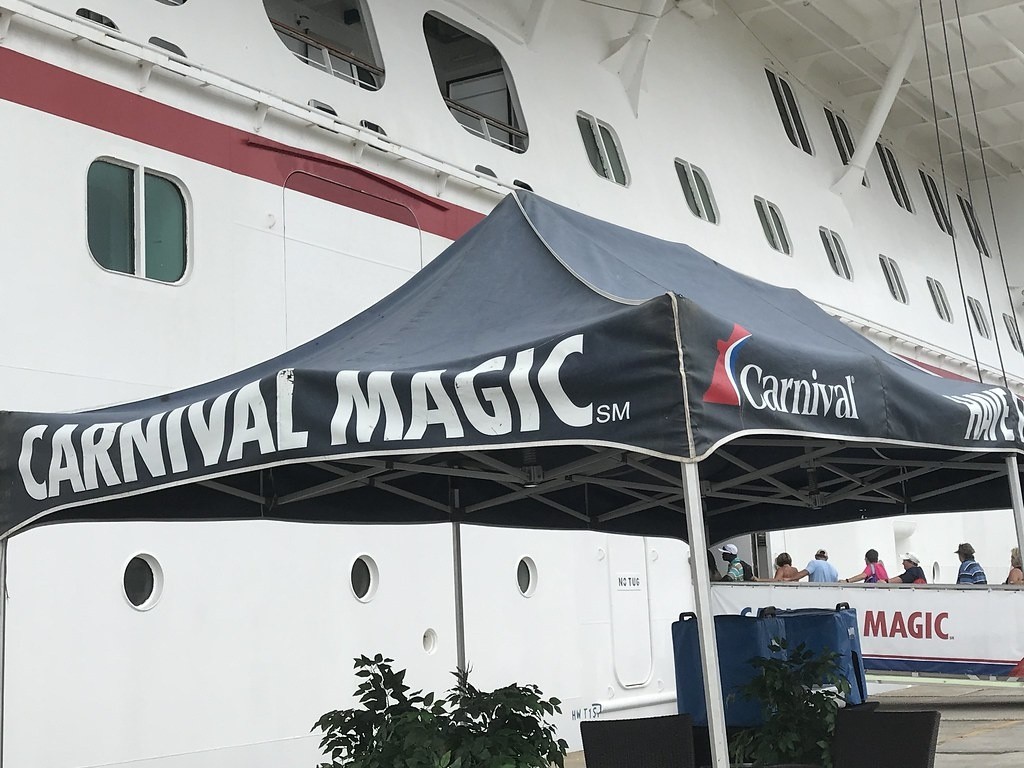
[735,560,753,581]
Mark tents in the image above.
[1,187,1024,767]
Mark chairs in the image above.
[833,707,941,768]
[580,713,697,768]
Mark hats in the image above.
[718,544,737,555]
[900,552,920,564]
[815,549,828,557]
[953,543,975,554]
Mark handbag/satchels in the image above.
[864,574,879,583]
[914,578,926,584]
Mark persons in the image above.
[954,542,988,591]
[1002,548,1024,591]
[876,552,930,590]
[837,548,890,589]
[707,550,717,585]
[777,549,837,587]
[750,552,799,587]
[717,543,745,586]
